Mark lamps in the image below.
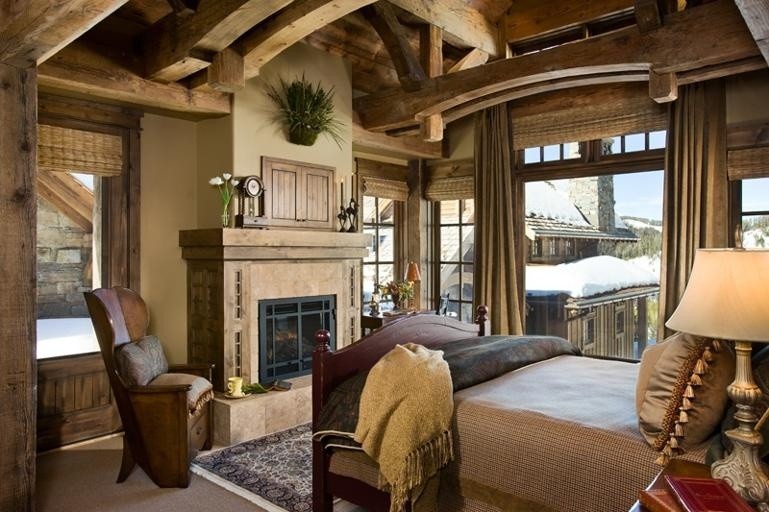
[404,261,421,311]
[664,224,769,505]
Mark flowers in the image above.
[374,279,417,305]
[208,173,240,226]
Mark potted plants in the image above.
[256,64,350,152]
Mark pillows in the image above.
[115,334,169,388]
[636,332,768,466]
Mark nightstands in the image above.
[628,458,712,512]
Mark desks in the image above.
[362,308,437,336]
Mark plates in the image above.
[223,391,252,399]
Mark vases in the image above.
[215,206,234,228]
[392,294,400,311]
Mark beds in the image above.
[312,306,769,512]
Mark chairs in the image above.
[435,293,449,316]
[83,285,215,488]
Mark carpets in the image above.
[188,419,342,512]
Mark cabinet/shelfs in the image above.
[261,156,336,231]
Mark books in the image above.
[639,488,683,512]
[664,474,756,512]
[262,379,292,391]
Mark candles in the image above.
[352,174,353,199]
[341,178,343,207]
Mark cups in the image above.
[227,377,243,396]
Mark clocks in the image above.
[237,175,269,229]
